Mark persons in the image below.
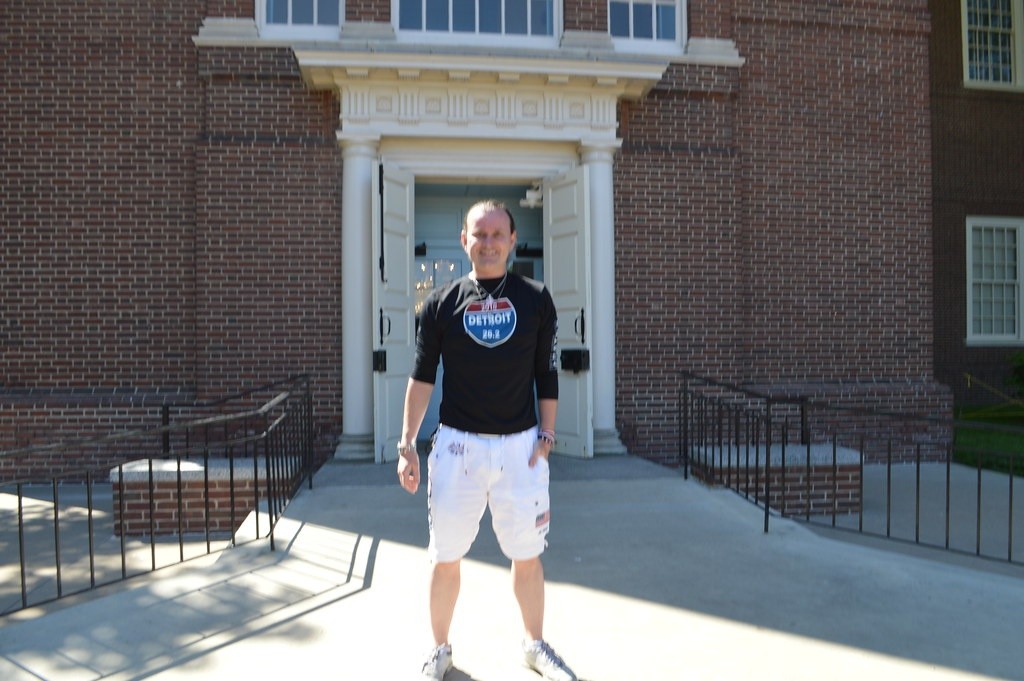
[396,199,577,681]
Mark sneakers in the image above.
[419,643,454,681]
[524,638,577,681]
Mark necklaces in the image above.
[473,270,507,315]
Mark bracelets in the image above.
[396,441,416,454]
[538,428,557,447]
